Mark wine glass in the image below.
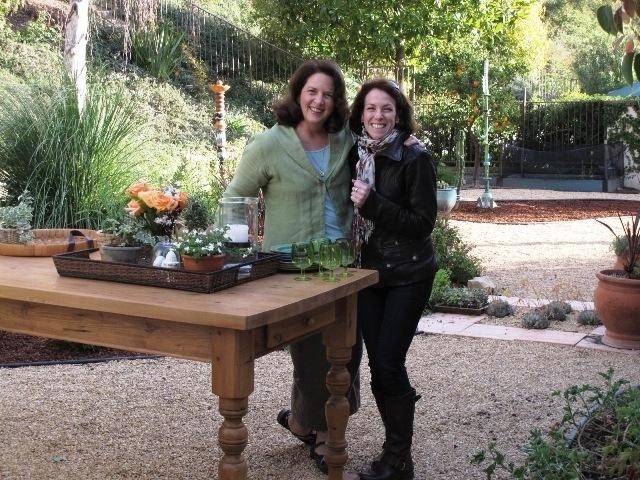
[289,237,356,284]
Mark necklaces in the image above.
[304,140,328,177]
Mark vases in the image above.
[154,235,178,259]
[181,248,227,272]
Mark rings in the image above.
[355,187,360,191]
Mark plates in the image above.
[270,241,318,271]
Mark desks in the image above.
[0,249,380,480]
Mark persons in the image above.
[223,61,427,474]
[348,78,438,480]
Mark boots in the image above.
[357,391,420,479]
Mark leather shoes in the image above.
[278,409,316,444]
[310,442,329,473]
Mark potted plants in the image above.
[96,218,155,264]
[432,284,492,315]
[0,201,35,245]
[593,203,640,350]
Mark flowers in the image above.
[123,178,189,236]
[174,224,233,261]
